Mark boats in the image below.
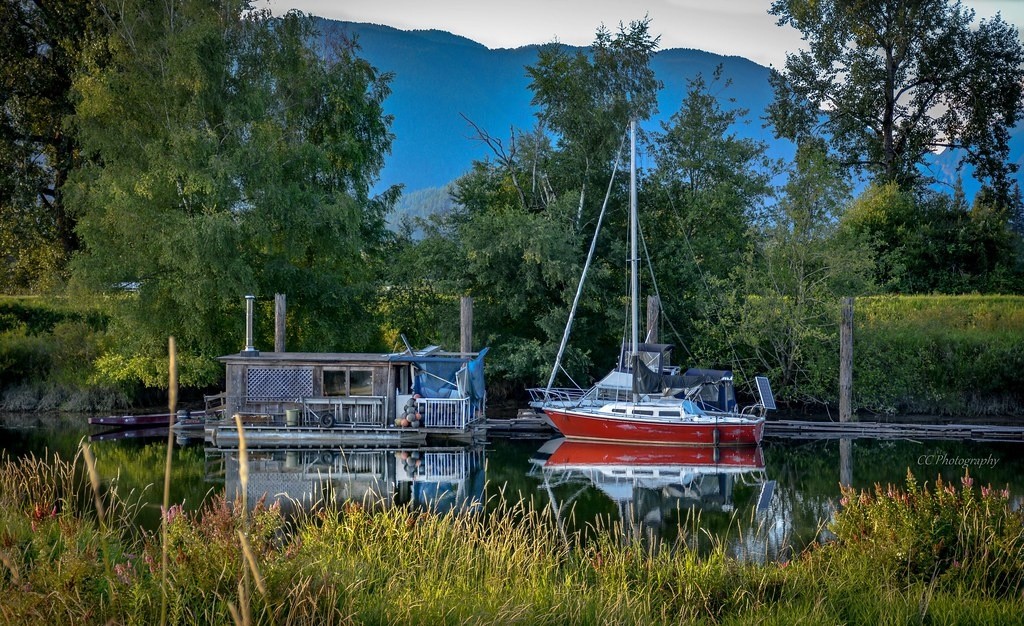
[525,120,774,446]
[525,436,766,503]
[89,425,175,442]
[88,407,229,438]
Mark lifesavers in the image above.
[319,412,335,428]
[318,450,335,466]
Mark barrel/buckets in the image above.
[285,409,299,427]
[285,452,297,468]
